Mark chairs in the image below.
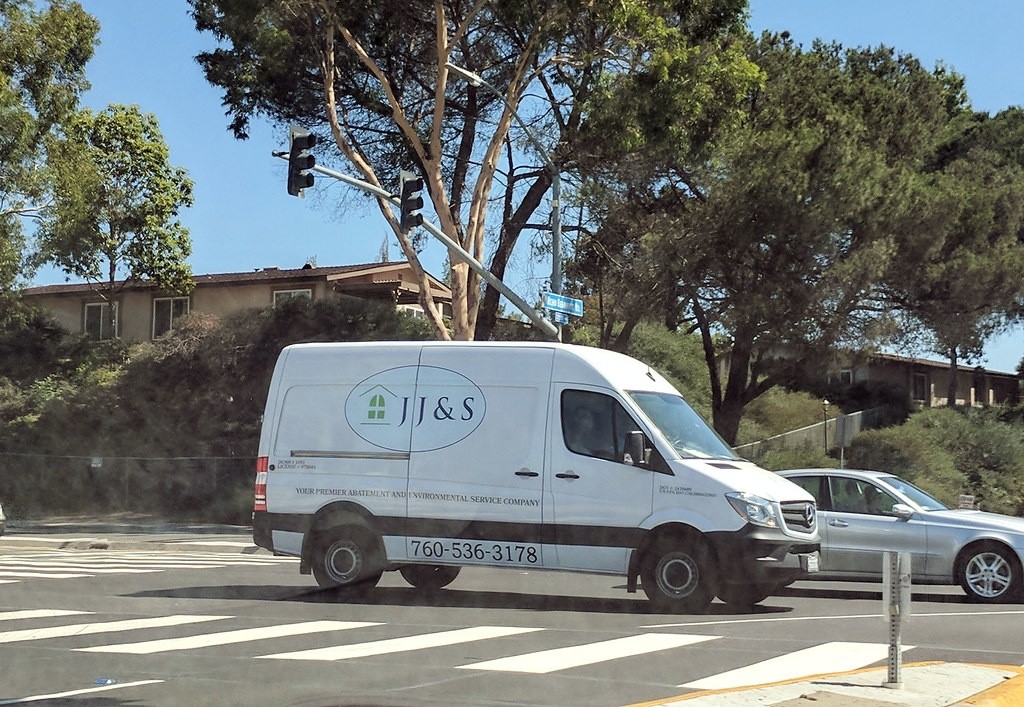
[831,479,846,511]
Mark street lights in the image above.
[445,62,564,343]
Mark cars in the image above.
[771,467,1024,601]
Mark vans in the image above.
[250,340,821,614]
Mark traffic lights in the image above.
[286,123,315,198]
[399,171,423,234]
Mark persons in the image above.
[860,485,886,515]
[572,408,651,465]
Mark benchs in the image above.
[564,406,605,453]
